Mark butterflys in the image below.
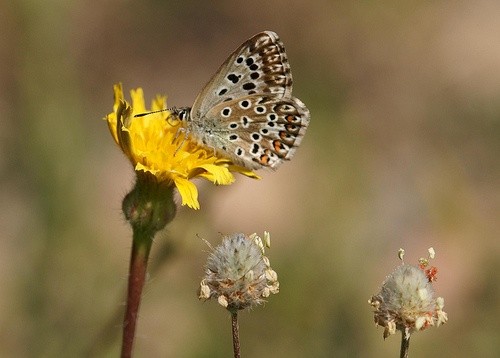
[133,30,311,173]
